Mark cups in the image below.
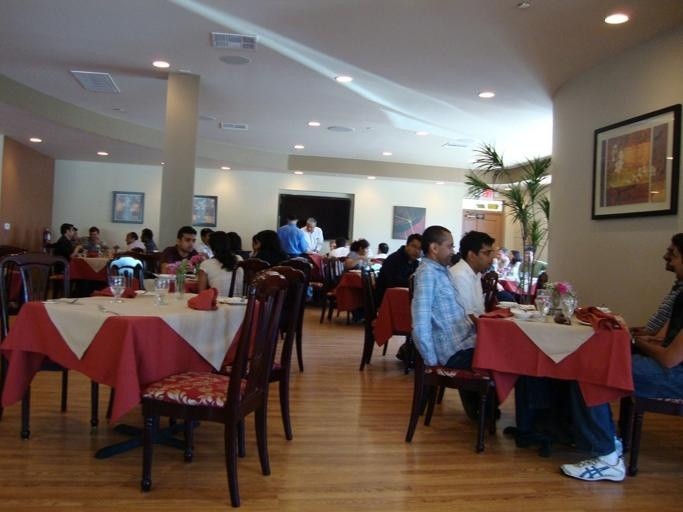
[560,294,577,319]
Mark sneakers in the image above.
[560,436,626,482]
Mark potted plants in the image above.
[462,137,551,306]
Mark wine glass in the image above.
[535,289,550,323]
[107,275,126,305]
[152,276,169,307]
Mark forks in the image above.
[45,297,80,304]
[97,300,122,318]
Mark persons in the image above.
[557,240,683,482]
[374,234,423,363]
[325,237,389,323]
[156,226,289,297]
[446,230,503,424]
[493,246,548,279]
[55,223,159,296]
[277,213,323,258]
[409,225,554,450]
[506,234,683,440]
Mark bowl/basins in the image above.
[508,308,532,320]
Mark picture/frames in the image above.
[191,195,217,227]
[590,103,680,221]
[110,190,145,225]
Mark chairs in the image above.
[619,382,683,476]
[312,247,409,371]
[403,270,495,453]
[0,231,311,505]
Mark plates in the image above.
[574,315,591,325]
[216,296,250,304]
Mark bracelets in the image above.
[630,335,637,347]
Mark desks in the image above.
[473,300,633,456]
[69,256,114,296]
[496,277,538,305]
[17,293,262,460]
